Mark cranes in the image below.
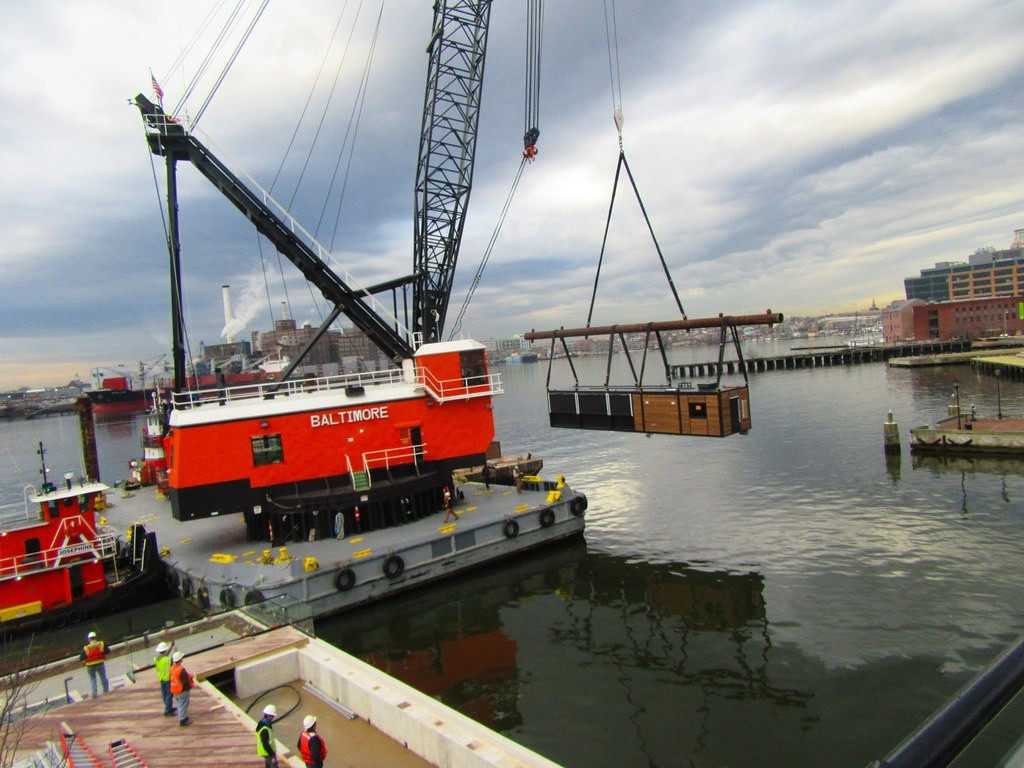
[135,0,623,548]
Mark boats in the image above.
[0,443,165,640]
[84,347,305,412]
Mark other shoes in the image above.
[164,708,177,715]
[180,718,194,726]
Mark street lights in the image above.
[952,378,962,429]
[995,369,1003,419]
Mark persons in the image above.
[79,632,111,698]
[296,715,326,768]
[154,641,194,727]
[442,486,459,522]
[482,464,495,490]
[971,404,976,419]
[255,704,278,768]
[513,466,524,494]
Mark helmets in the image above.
[263,705,277,716]
[156,642,169,652]
[303,715,316,729]
[173,652,184,662]
[87,632,97,638]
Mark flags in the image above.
[151,74,164,106]
